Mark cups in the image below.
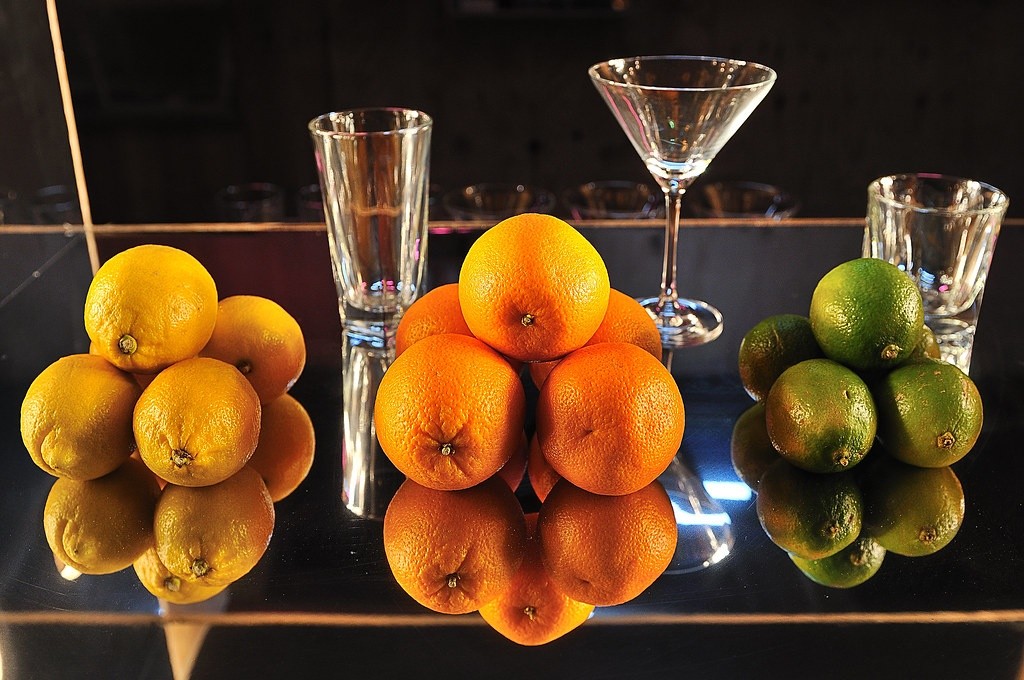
[862,174,1010,341]
[935,335,973,377]
[341,329,407,521]
[309,107,433,343]
[210,182,785,221]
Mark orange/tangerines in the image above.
[373,214,686,497]
[381,434,677,646]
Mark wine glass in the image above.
[658,340,735,575]
[589,56,777,340]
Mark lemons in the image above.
[21,245,306,488]
[42,394,317,604]
[730,401,965,588]
[736,257,984,475]
